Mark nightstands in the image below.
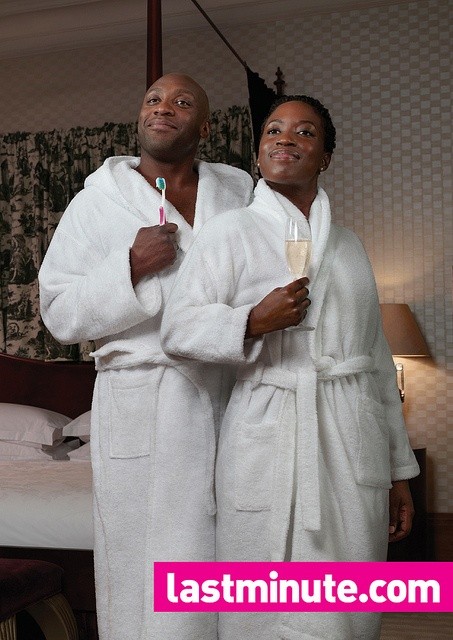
[389,448,427,560]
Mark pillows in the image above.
[0,402,73,446]
[66,442,92,464]
[63,409,91,443]
[0,439,80,460]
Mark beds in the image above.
[0,353,96,552]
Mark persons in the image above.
[159,94,422,639]
[37,72,255,639]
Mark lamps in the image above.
[380,303,432,403]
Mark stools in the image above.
[0,558,78,639]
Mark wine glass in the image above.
[283,217,315,333]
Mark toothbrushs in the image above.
[155,176,167,226]
